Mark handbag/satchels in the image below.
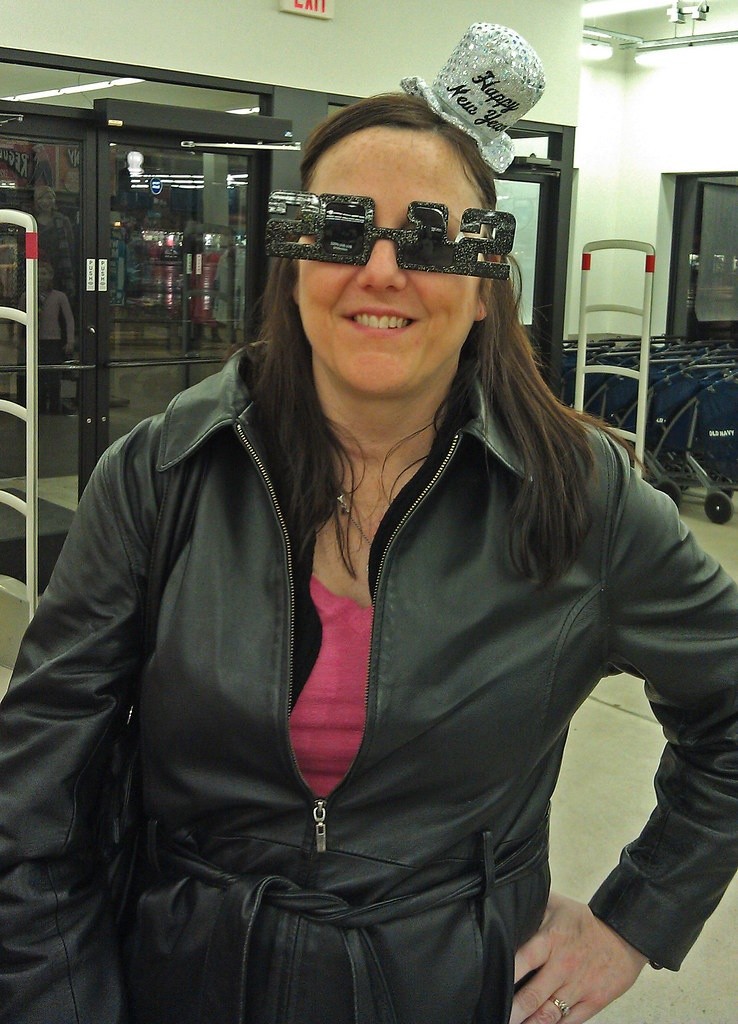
[101,436,216,934]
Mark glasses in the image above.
[261,190,517,280]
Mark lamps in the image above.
[581,38,613,61]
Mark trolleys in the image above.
[563,332,738,524]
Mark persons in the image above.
[17,261,75,415]
[31,181,76,302]
[0,21,738,1024]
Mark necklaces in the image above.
[337,493,371,546]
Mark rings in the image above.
[548,996,569,1016]
